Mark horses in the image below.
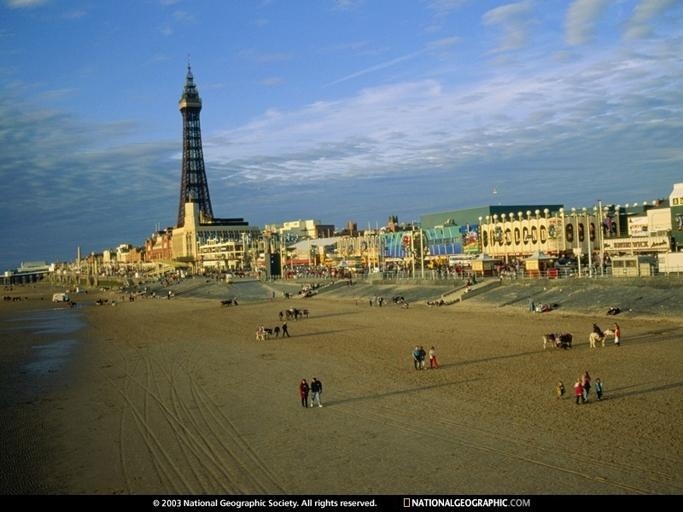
[296,309,309,318]
[279,309,295,320]
[542,333,559,350]
[589,328,615,348]
[256,328,272,340]
[559,333,572,347]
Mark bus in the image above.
[447,254,472,267]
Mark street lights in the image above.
[415,229,426,280]
[478,200,659,280]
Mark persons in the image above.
[412,345,439,369]
[427,277,477,306]
[555,321,620,405]
[300,379,309,408]
[310,378,324,408]
[255,271,404,341]
[528,298,558,312]
